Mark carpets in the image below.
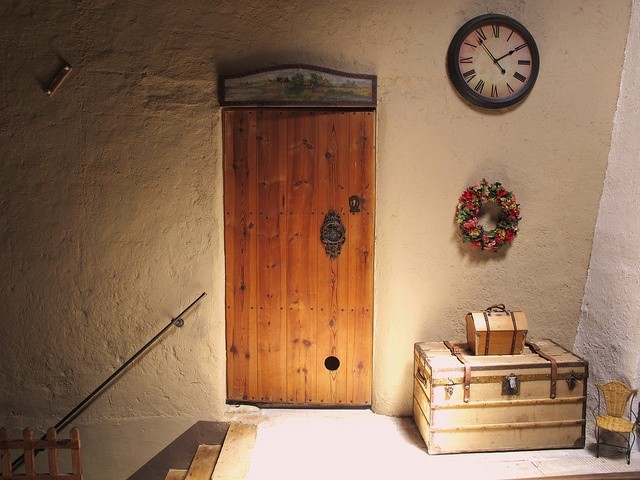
[246,421,640,480]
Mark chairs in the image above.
[593,381,638,466]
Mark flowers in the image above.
[456,180,522,250]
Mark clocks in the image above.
[446,14,540,108]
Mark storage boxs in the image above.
[466,303,527,355]
[414,338,589,454]
